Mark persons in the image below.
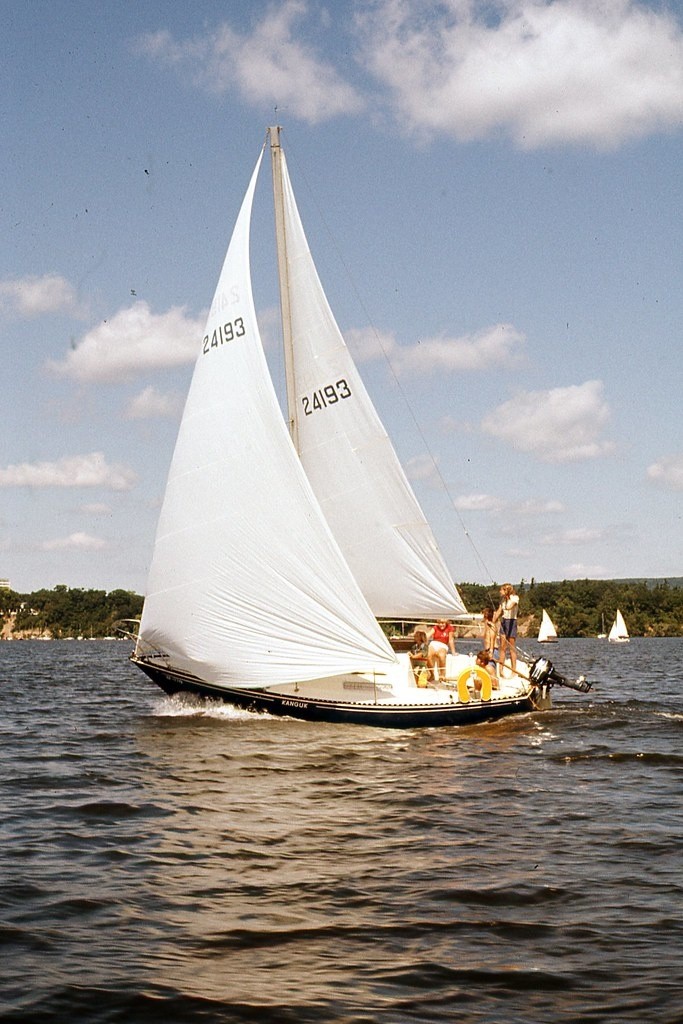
[477,650,500,691]
[409,628,428,685]
[426,618,459,684]
[482,605,497,662]
[493,584,519,677]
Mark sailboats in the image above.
[116,96,594,730]
